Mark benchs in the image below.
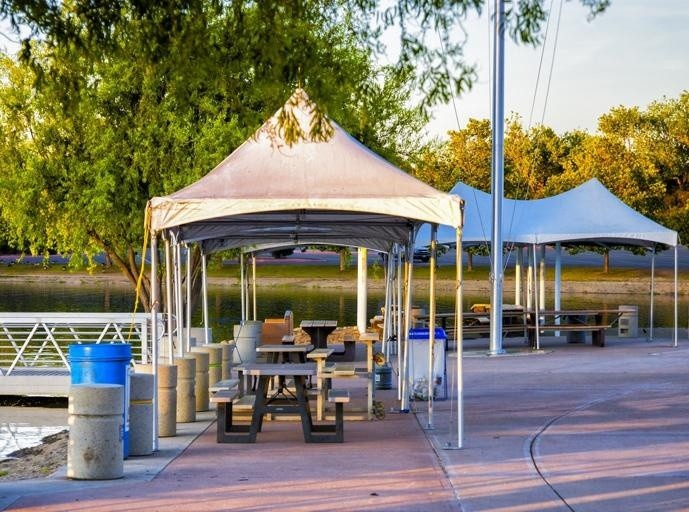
[209,387,250,441]
[369,307,636,350]
[213,309,380,421]
[327,388,351,441]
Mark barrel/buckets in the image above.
[233,324,259,363]
[617,306,640,337]
[68,344,133,460]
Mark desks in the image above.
[242,362,318,439]
